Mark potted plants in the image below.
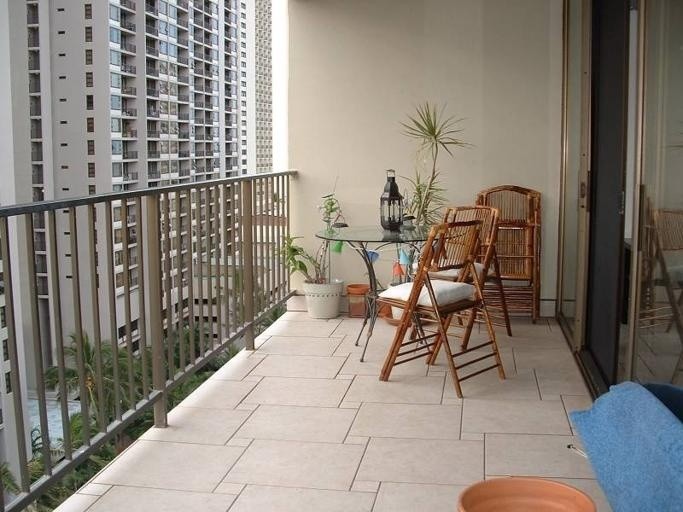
[267,193,348,319]
[384,97,476,326]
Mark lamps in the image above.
[380,169,404,231]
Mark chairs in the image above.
[375,184,541,399]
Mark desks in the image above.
[314,224,466,363]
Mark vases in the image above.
[347,282,370,318]
[456,474,597,512]
